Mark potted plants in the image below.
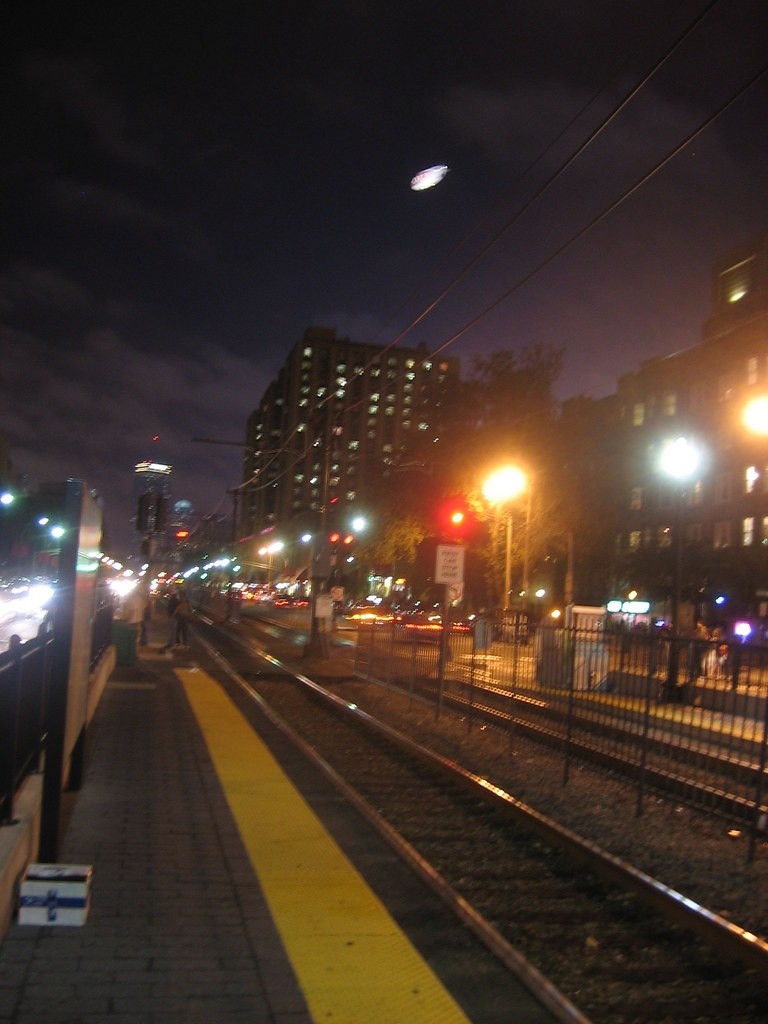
[15,860,95,927]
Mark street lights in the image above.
[658,433,696,701]
[486,463,533,623]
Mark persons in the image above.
[169,588,193,645]
[139,598,154,645]
[695,622,728,679]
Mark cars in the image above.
[228,582,473,644]
